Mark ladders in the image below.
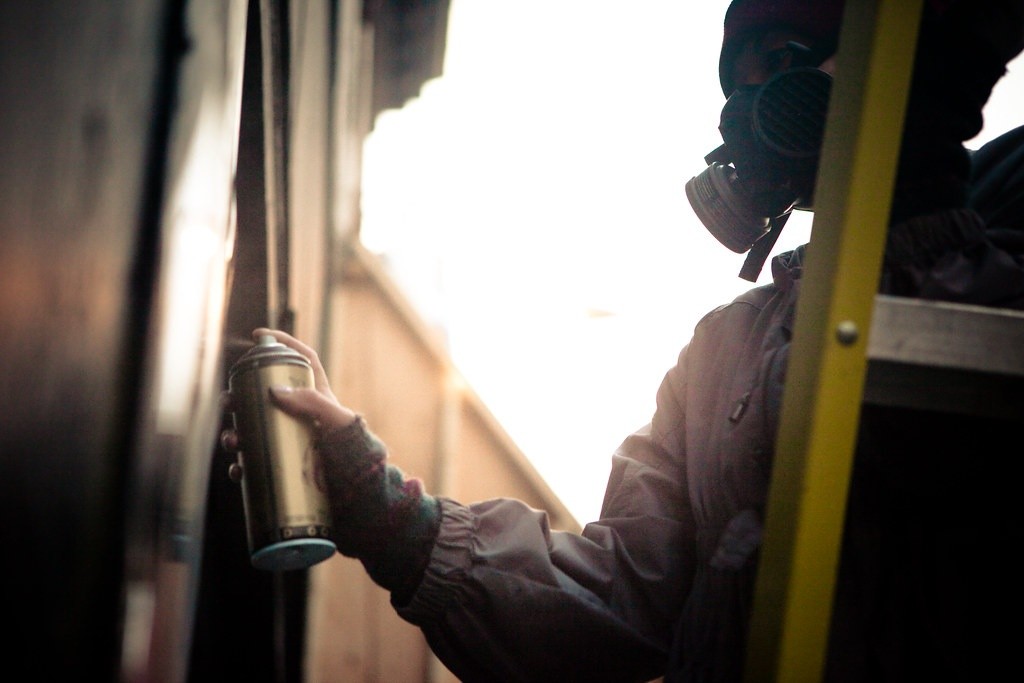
[733,1,1024,683]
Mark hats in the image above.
[718,0,1023,139]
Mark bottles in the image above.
[230,336,337,568]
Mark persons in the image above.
[217,0,1024,683]
